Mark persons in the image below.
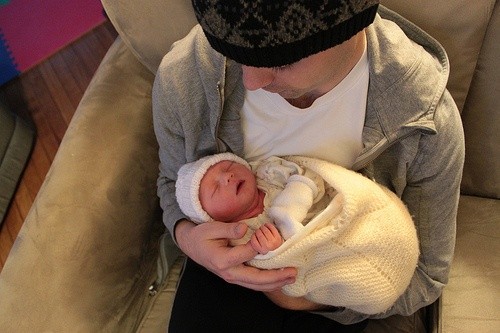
[175,152,419,314]
[151,0,465,333]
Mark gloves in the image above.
[267,206,306,243]
[269,174,318,222]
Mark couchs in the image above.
[0,0,500,333]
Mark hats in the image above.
[174,151,254,226]
[191,0,381,68]
